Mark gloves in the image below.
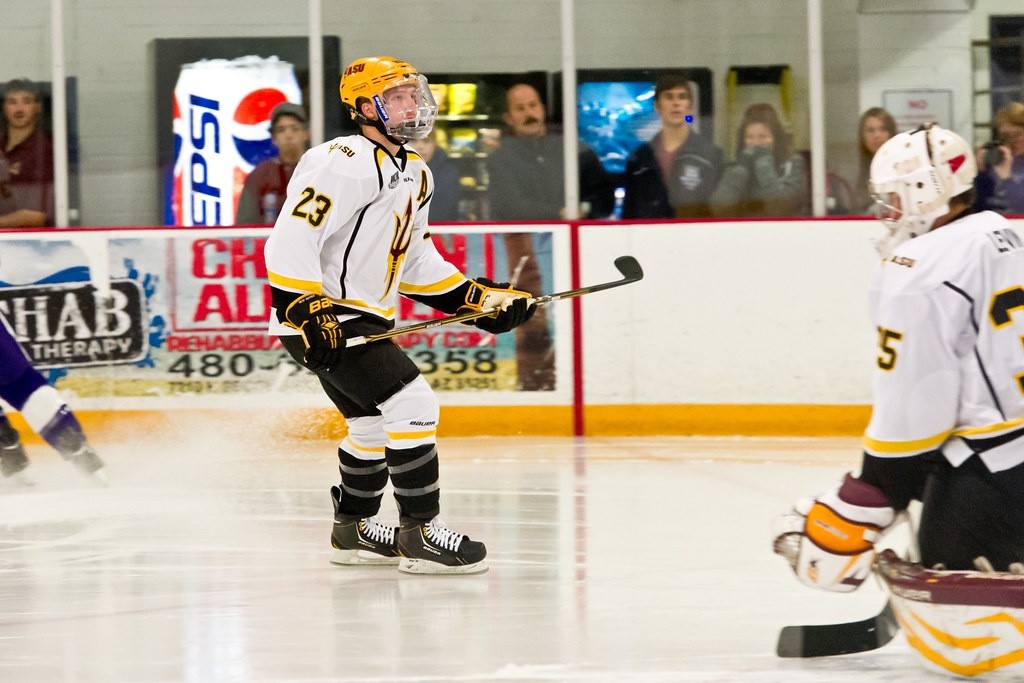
[281,294,346,372]
[455,277,537,335]
[793,473,895,595]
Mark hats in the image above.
[270,103,306,125]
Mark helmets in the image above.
[869,121,977,257]
[340,55,437,140]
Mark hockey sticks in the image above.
[341,255,644,349]
[775,487,936,659]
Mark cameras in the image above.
[985,140,1004,166]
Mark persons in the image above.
[971,102,1024,216]
[775,120,1024,683]
[0,77,55,227]
[707,101,806,218]
[0,308,112,490]
[621,74,723,218]
[262,55,536,577]
[487,83,617,222]
[235,102,310,226]
[854,106,897,217]
[404,128,461,221]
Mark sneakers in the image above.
[330,486,400,566]
[55,428,111,483]
[393,490,490,574]
[0,417,37,485]
